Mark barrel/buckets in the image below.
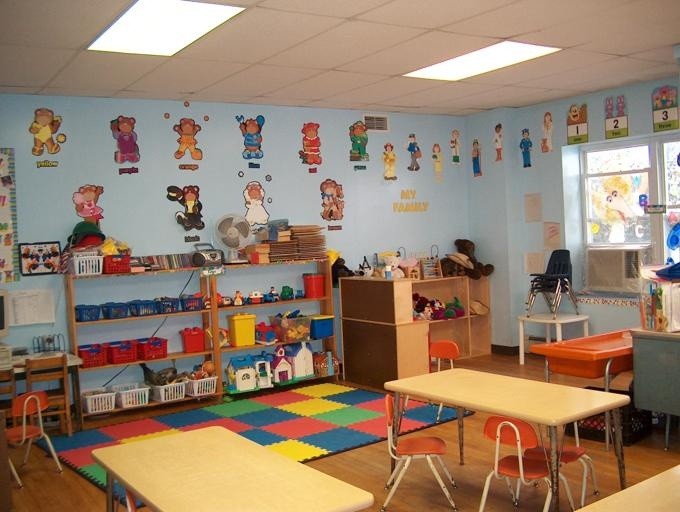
[302,272,326,297]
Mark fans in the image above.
[217,215,254,265]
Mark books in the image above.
[128,252,193,271]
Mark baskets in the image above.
[77,342,108,369]
[129,299,157,317]
[76,303,101,323]
[137,337,170,360]
[80,386,116,414]
[181,294,203,312]
[112,382,151,409]
[563,384,655,448]
[108,339,138,366]
[158,296,180,315]
[104,302,128,319]
[183,373,219,398]
[147,376,189,403]
[66,250,132,276]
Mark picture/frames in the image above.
[18,243,60,276]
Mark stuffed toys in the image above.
[412,292,465,321]
[326,239,494,287]
[189,357,215,380]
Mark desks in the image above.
[579,467,680,512]
[90,426,377,512]
[530,330,633,452]
[629,325,680,452]
[383,368,630,511]
[498,364,633,488]
[1,350,86,430]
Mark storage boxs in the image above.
[179,274,337,354]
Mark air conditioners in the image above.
[584,247,652,295]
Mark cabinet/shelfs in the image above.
[64,263,222,429]
[214,253,337,396]
[339,268,490,393]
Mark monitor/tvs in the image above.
[0,290,10,338]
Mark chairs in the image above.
[518,309,589,365]
[478,412,577,511]
[1,369,18,428]
[524,247,581,320]
[524,440,605,502]
[18,357,74,440]
[383,388,457,512]
[4,390,64,489]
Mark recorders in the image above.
[189,243,225,267]
